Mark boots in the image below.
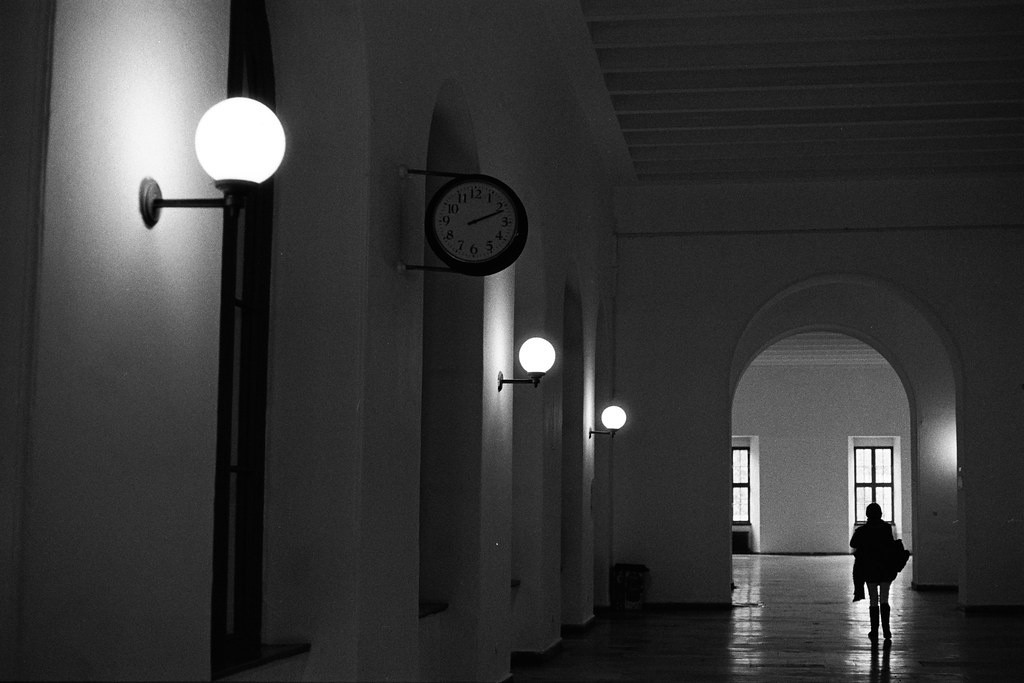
[869,606,880,640]
[881,606,891,638]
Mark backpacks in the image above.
[881,520,910,580]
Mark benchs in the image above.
[211,641,310,681]
[420,596,447,619]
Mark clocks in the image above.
[425,174,529,277]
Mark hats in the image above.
[866,503,882,517]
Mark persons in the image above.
[850,503,910,640]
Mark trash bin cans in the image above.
[610,561,652,620]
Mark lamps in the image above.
[589,406,627,439]
[139,98,287,229]
[498,337,556,392]
